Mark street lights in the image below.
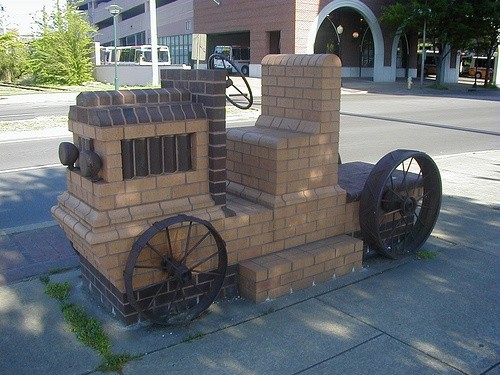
[105,5,123,92]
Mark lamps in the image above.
[326,15,343,33]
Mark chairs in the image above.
[225,54,345,208]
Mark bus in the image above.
[213,45,280,76]
[460,56,494,79]
[100,45,172,66]
[417,51,440,75]
